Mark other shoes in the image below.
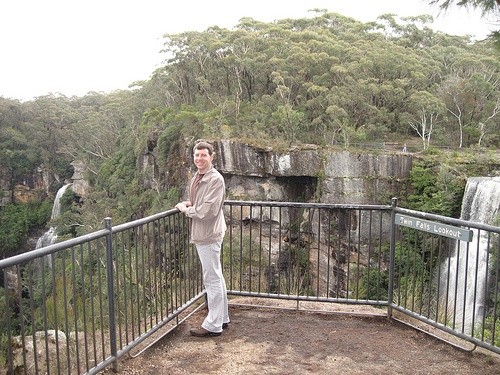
[221,323,228,329]
[190,326,222,337]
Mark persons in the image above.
[175,142,231,337]
